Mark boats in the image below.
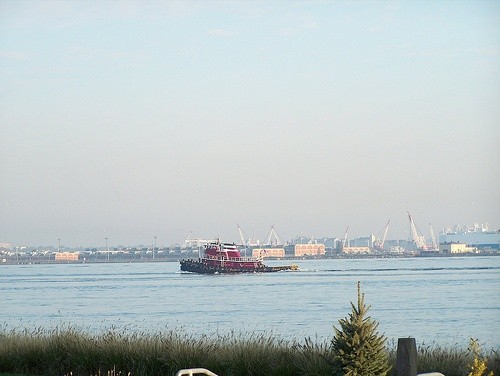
[180,238,299,274]
